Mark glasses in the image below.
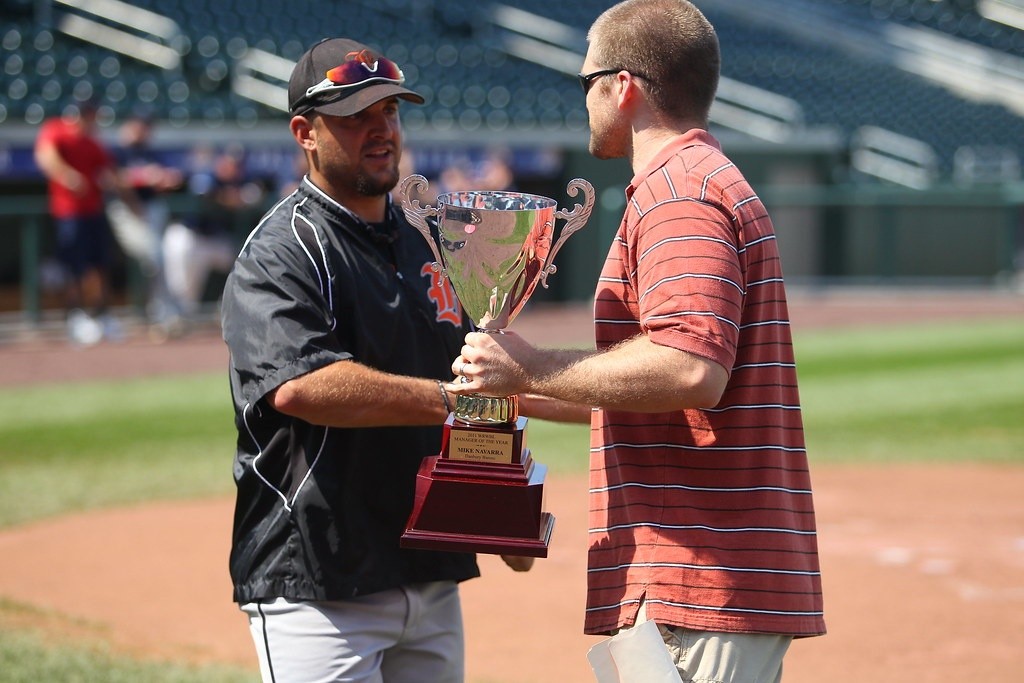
[580,68,656,92]
[305,61,406,98]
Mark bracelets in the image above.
[434,378,453,415]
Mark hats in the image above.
[287,38,425,117]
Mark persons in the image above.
[161,138,247,335]
[445,0,828,683]
[34,85,126,343]
[104,109,176,339]
[218,38,518,683]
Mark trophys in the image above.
[398,175,595,559]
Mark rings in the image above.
[458,362,469,377]
